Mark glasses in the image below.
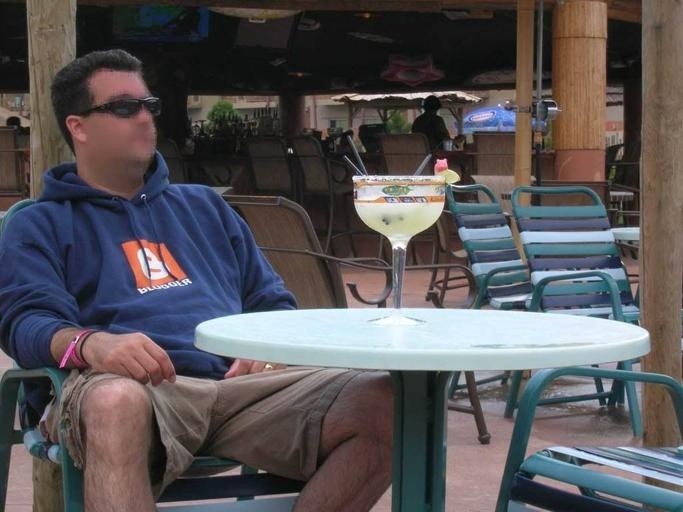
[75,95,162,119]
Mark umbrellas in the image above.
[453,105,548,137]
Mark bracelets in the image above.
[80,331,102,365]
[59,329,87,368]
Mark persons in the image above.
[7,117,29,135]
[0,49,392,512]
[411,96,453,150]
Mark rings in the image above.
[265,364,273,369]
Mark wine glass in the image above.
[353,176,447,328]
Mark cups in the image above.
[444,139,454,152]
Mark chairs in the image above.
[159,121,683,265]
[0,198,682,512]
